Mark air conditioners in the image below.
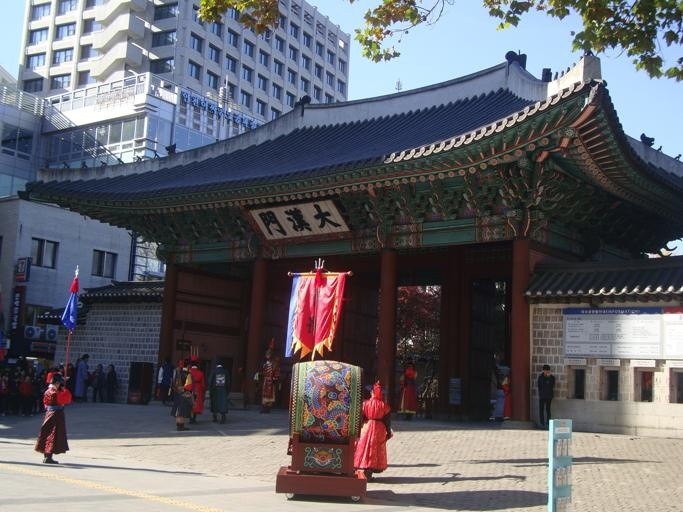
[45,323,59,344]
[24,325,40,341]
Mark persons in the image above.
[487,352,512,420]
[395,363,420,422]
[253,348,280,415]
[154,353,232,433]
[0,353,120,420]
[351,379,393,482]
[536,363,555,431]
[33,370,73,465]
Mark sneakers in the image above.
[44,458,58,463]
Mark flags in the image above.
[60,278,78,335]
[283,271,353,361]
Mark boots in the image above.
[260,404,271,414]
[177,406,226,432]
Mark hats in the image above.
[49,372,69,384]
